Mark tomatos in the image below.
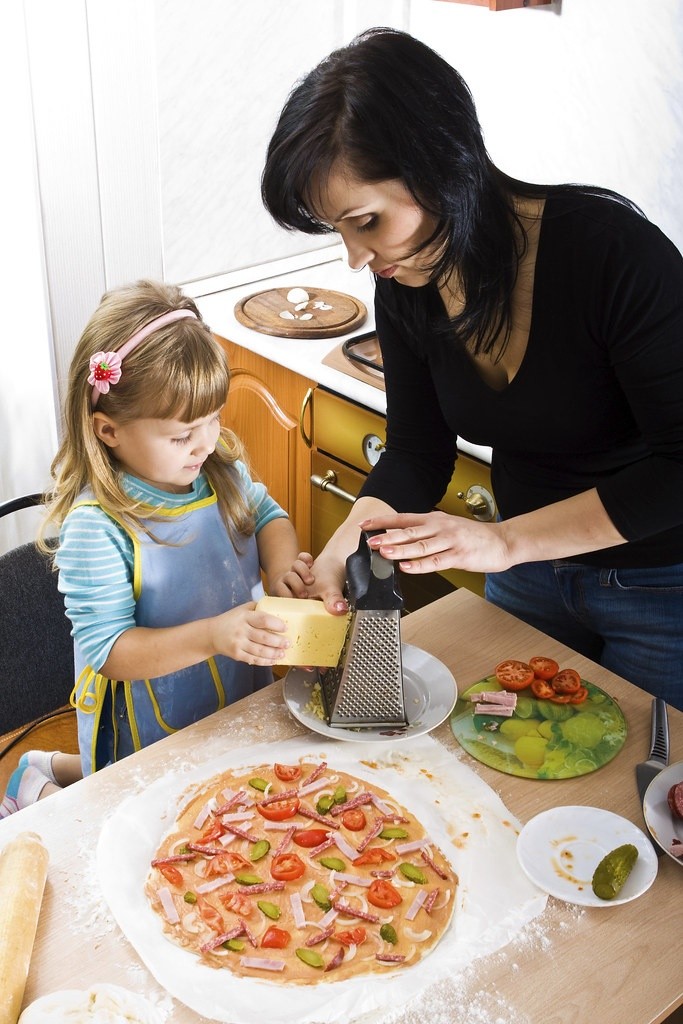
[495,657,588,703]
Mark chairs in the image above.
[0,490,85,819]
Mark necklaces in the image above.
[444,273,466,306]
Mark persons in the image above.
[0,279,313,820]
[259,26,683,713]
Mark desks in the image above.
[0,586,683,1024]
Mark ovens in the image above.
[310,382,495,604]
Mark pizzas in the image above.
[141,763,461,985]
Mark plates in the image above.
[283,642,458,742]
[517,805,658,907]
[643,757,683,868]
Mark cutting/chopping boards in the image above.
[233,285,367,340]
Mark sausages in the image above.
[591,844,638,899]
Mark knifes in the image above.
[636,697,670,857]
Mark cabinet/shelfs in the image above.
[215,335,317,558]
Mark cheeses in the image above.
[252,596,351,667]
